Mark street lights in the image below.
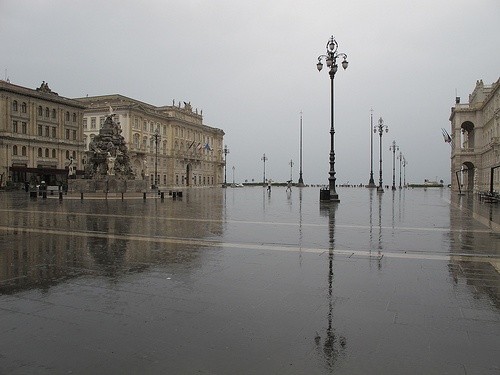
[288,159,295,182]
[397,152,408,189]
[153,133,161,188]
[221,144,231,189]
[260,153,268,187]
[389,140,401,191]
[373,116,389,193]
[315,35,349,205]
[231,166,235,185]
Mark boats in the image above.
[402,175,445,188]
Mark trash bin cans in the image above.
[320,187,330,201]
[30,192,37,198]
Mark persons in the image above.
[267,178,272,193]
[286,179,292,192]
[66,154,74,178]
[107,152,117,176]
[25,180,30,193]
[425,181,428,188]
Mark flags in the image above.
[197,143,202,150]
[442,130,451,143]
